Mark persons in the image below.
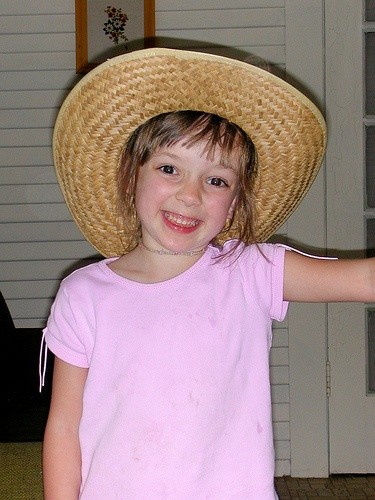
[37,109,375,500]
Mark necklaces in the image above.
[138,237,209,257]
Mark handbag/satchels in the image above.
[0,292,55,443]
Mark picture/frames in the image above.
[74,0,156,77]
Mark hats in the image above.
[51,45,328,258]
